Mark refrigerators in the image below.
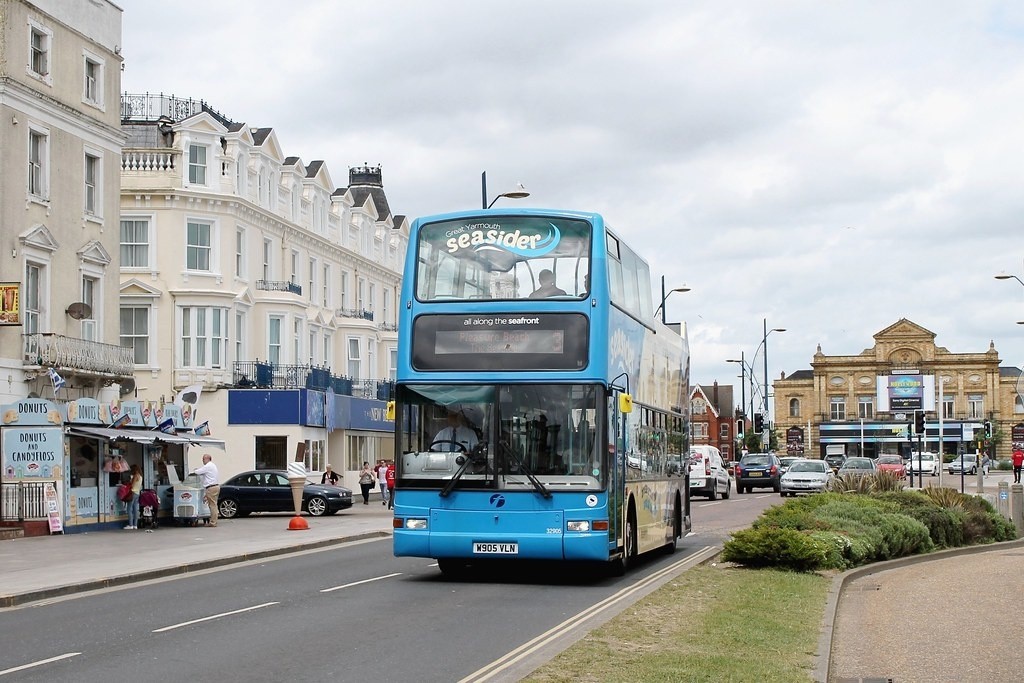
[173,488,219,518]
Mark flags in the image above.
[49,368,65,396]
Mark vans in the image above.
[688,445,733,501]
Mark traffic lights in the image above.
[737,420,745,439]
[914,410,925,435]
[985,422,992,437]
[753,412,764,434]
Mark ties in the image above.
[450,428,456,452]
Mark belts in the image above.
[206,484,219,489]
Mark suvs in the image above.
[736,451,788,494]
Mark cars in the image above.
[823,453,847,474]
[780,460,835,498]
[906,453,941,478]
[780,456,803,469]
[726,461,739,475]
[837,457,877,481]
[875,454,907,481]
[948,454,989,476]
[217,470,356,519]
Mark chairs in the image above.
[815,465,822,471]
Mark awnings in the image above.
[66,426,225,450]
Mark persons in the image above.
[321,464,339,486]
[124,465,143,529]
[563,437,589,476]
[1012,447,1024,483]
[187,454,220,527]
[578,275,589,298]
[385,459,396,510]
[529,269,567,297]
[430,403,478,455]
[359,462,375,504]
[373,459,388,505]
[983,452,990,478]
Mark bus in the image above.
[386,209,691,576]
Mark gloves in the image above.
[188,472,196,476]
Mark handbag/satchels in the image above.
[103,458,114,473]
[118,482,132,502]
[112,457,121,472]
[120,456,130,472]
[370,480,375,489]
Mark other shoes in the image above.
[364,501,368,504]
[390,505,394,510]
[383,501,385,505]
[133,526,137,529]
[123,526,133,530]
[204,523,216,527]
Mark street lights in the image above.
[749,329,787,424]
[652,287,689,321]
[726,359,746,451]
[480,190,529,299]
